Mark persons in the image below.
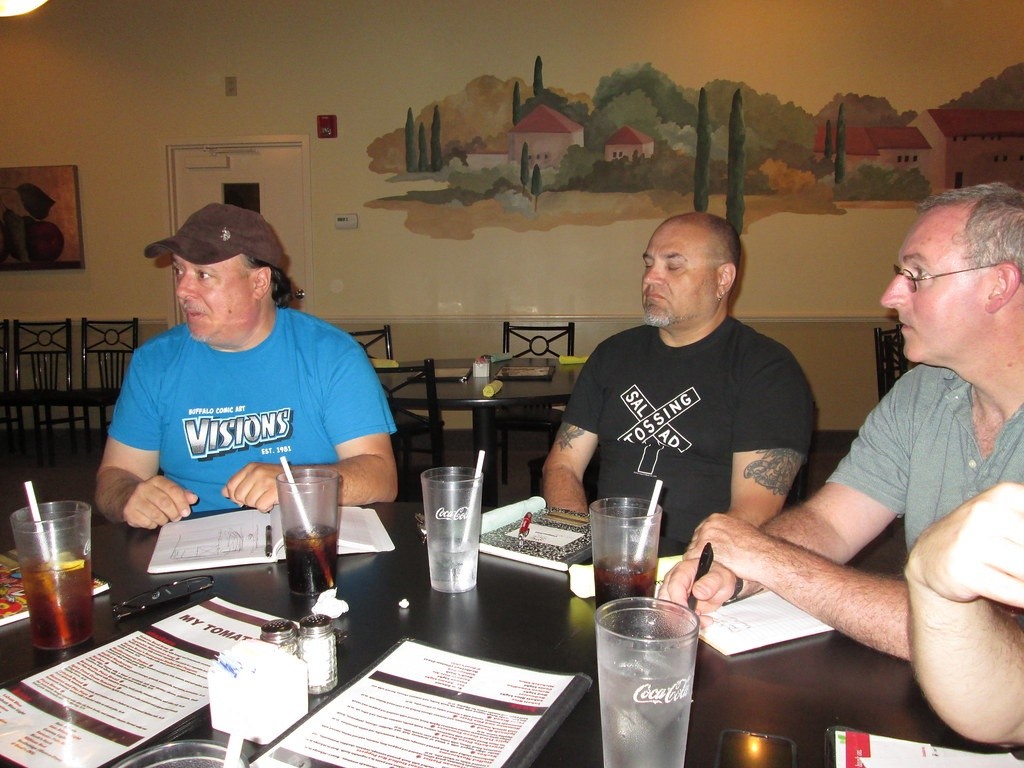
[905,483,1024,747]
[542,214,815,558]
[95,203,398,528]
[659,181,1024,659]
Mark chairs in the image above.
[348,324,444,503]
[494,321,600,507]
[0,317,138,468]
[874,325,906,401]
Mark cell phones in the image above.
[713,728,796,768]
[823,725,935,768]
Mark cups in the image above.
[111,740,250,768]
[594,597,700,768]
[422,467,483,592]
[588,497,662,610]
[11,501,93,649]
[276,468,340,595]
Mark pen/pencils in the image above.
[515,511,533,553]
[265,524,277,561]
[689,543,714,612]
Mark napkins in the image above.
[558,356,588,364]
[491,353,513,362]
[447,497,546,538]
[568,555,684,598]
[370,358,399,367]
[481,381,503,397]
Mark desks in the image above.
[0,501,1023,768]
[377,357,586,508]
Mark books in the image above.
[569,556,836,655]
[479,507,619,572]
[0,548,111,626]
[146,504,395,574]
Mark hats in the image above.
[144,203,285,271]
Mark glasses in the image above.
[893,263,1024,292]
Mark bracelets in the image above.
[721,577,743,606]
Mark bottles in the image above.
[260,618,298,659]
[298,614,338,694]
